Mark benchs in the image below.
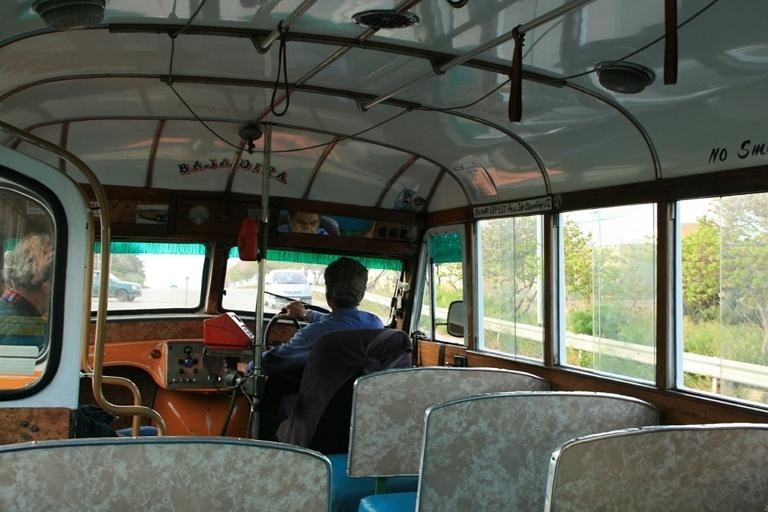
[356,388,662,512]
[3,433,337,512]
[319,368,556,512]
[539,420,767,512]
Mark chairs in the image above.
[286,328,413,456]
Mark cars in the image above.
[258,270,314,312]
[91,270,143,303]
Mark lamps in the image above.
[32,0,105,29]
[594,58,654,94]
[352,9,419,30]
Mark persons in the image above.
[277,211,329,235]
[237,257,384,395]
[0,233,119,439]
